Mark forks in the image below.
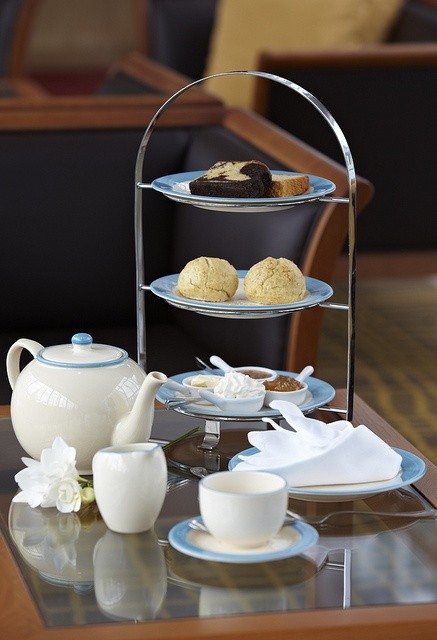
[186,518,297,534]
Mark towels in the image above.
[232,400,403,488]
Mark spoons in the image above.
[285,509,436,525]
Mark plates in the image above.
[227,446,427,503]
[154,368,336,417]
[149,269,332,320]
[151,170,336,213]
[167,515,319,564]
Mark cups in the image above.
[91,442,168,535]
[91,535,166,621]
[197,470,288,548]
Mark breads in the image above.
[272,175,310,197]
[178,256,238,302]
[244,256,306,305]
[189,159,272,197]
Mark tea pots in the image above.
[5,477,104,596]
[5,332,167,476]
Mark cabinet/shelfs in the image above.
[134,66,359,479]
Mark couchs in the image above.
[0,105,373,394]
[2,57,223,107]
[254,43,433,256]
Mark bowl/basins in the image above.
[198,389,265,412]
[259,365,314,408]
[209,355,276,383]
[160,375,223,397]
